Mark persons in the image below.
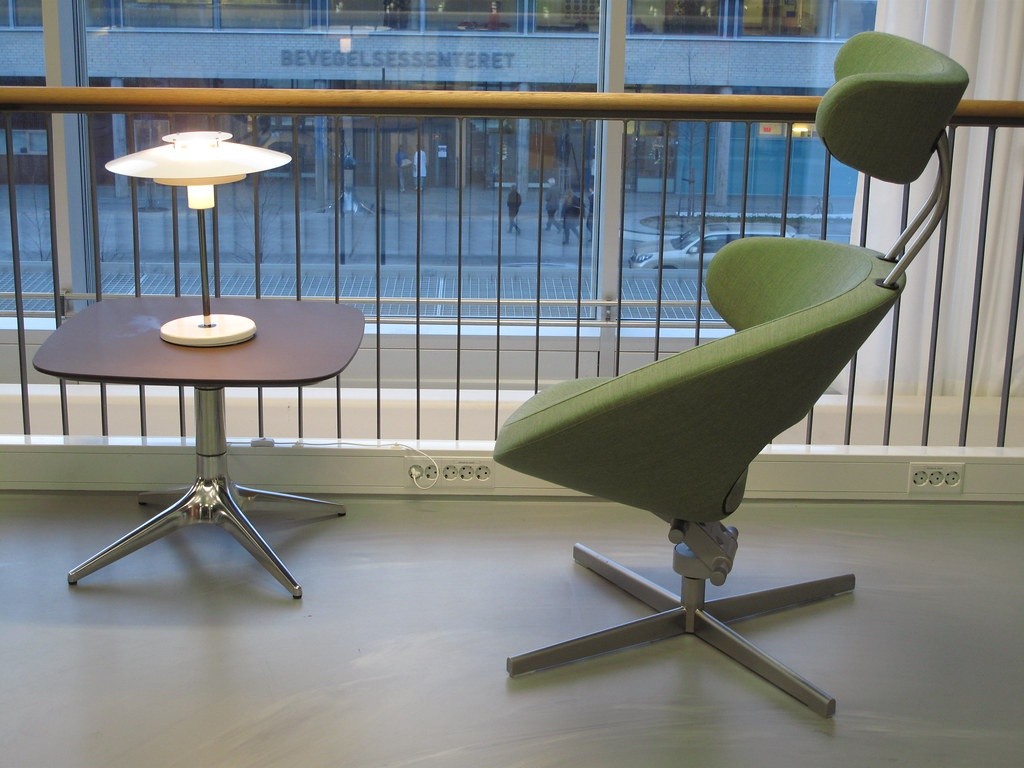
[507,185,521,235]
[395,145,408,192]
[413,145,426,192]
[545,178,562,233]
[633,18,647,33]
[586,186,594,241]
[560,190,579,245]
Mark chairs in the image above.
[492,30,971,718]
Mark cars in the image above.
[629,222,799,271]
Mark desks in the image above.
[32,297,366,598]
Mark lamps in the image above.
[105,131,293,347]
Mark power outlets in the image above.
[909,462,965,495]
[404,455,494,488]
